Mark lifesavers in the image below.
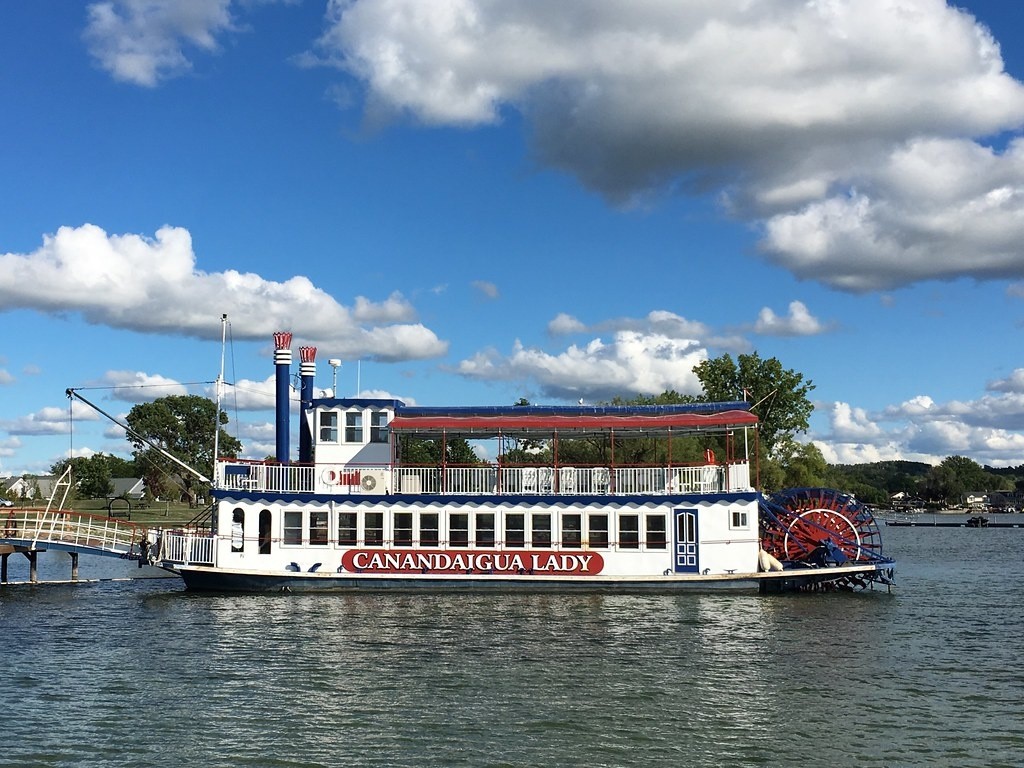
[5,514,17,536]
[321,466,340,486]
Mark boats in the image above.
[64,312,902,600]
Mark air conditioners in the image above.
[360,470,386,495]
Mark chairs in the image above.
[521,467,537,492]
[700,466,719,490]
[591,467,611,493]
[665,471,679,492]
[538,467,554,492]
[561,467,577,494]
